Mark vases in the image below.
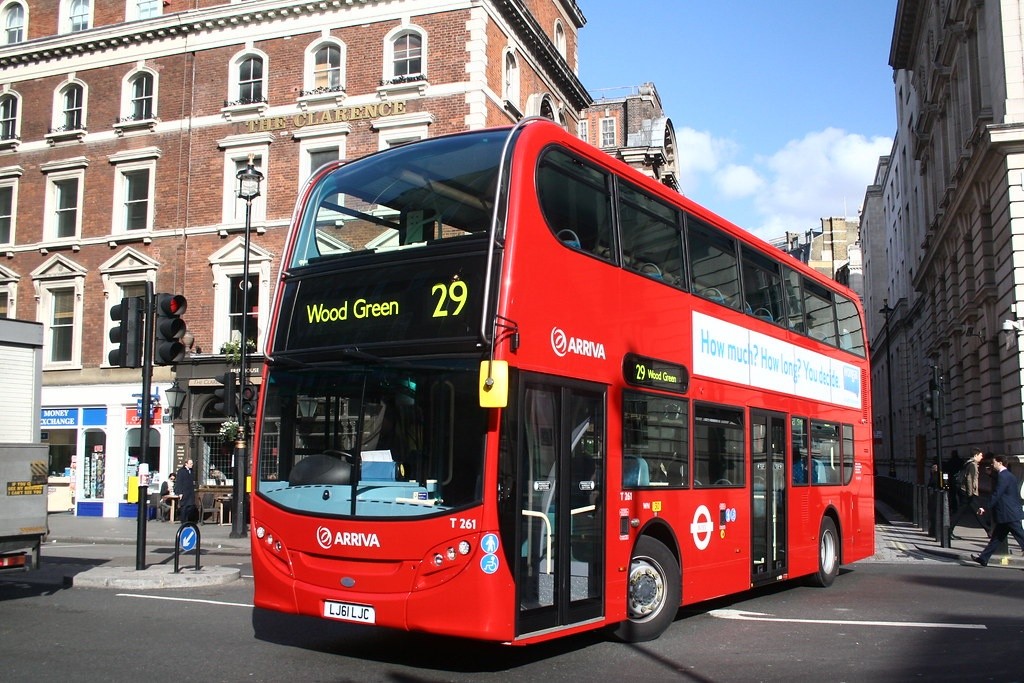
[221,347,256,354]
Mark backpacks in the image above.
[953,460,974,487]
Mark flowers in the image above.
[223,339,256,364]
[217,420,239,443]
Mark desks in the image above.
[163,496,179,523]
[212,497,232,526]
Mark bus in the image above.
[248,115,875,646]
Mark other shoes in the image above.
[971,554,987,567]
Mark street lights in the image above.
[230,156,265,539]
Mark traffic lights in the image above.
[153,292,188,366]
[108,296,145,368]
[242,384,257,417]
[214,372,236,416]
[925,389,940,419]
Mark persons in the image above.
[518,450,601,561]
[926,445,1024,566]
[160,458,196,526]
[790,446,803,485]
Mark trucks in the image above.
[0,316,50,572]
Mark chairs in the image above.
[198,497,220,525]
[147,493,161,521]
[621,453,855,534]
[557,229,845,351]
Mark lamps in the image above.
[165,377,187,420]
[966,326,985,339]
